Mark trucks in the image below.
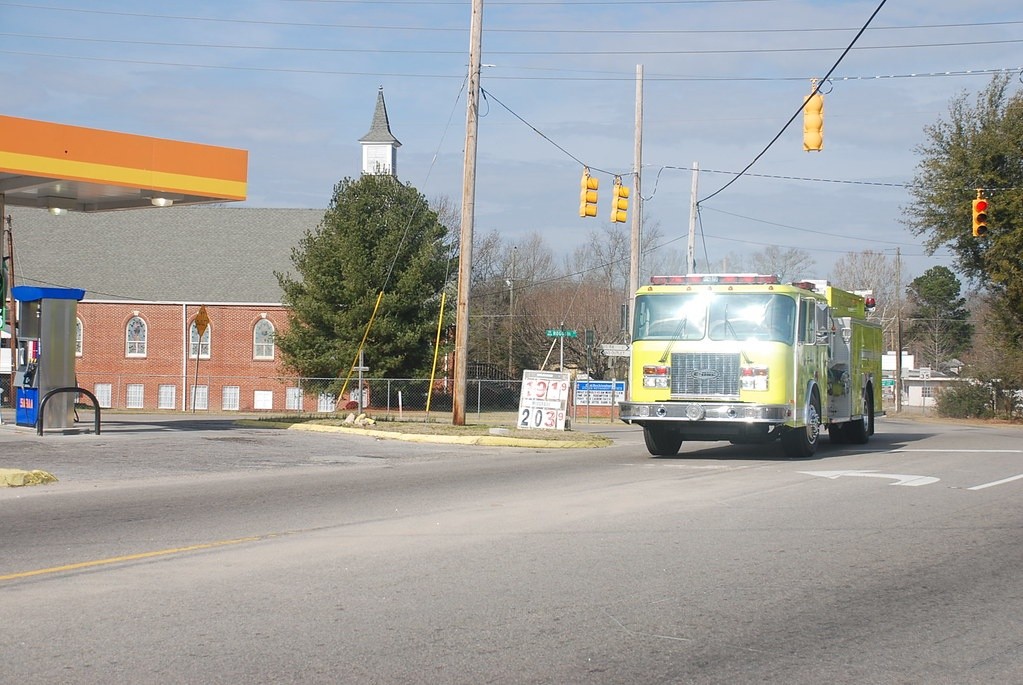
[617,276,885,456]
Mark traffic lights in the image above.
[578,169,601,218]
[972,198,990,237]
[612,185,628,225]
